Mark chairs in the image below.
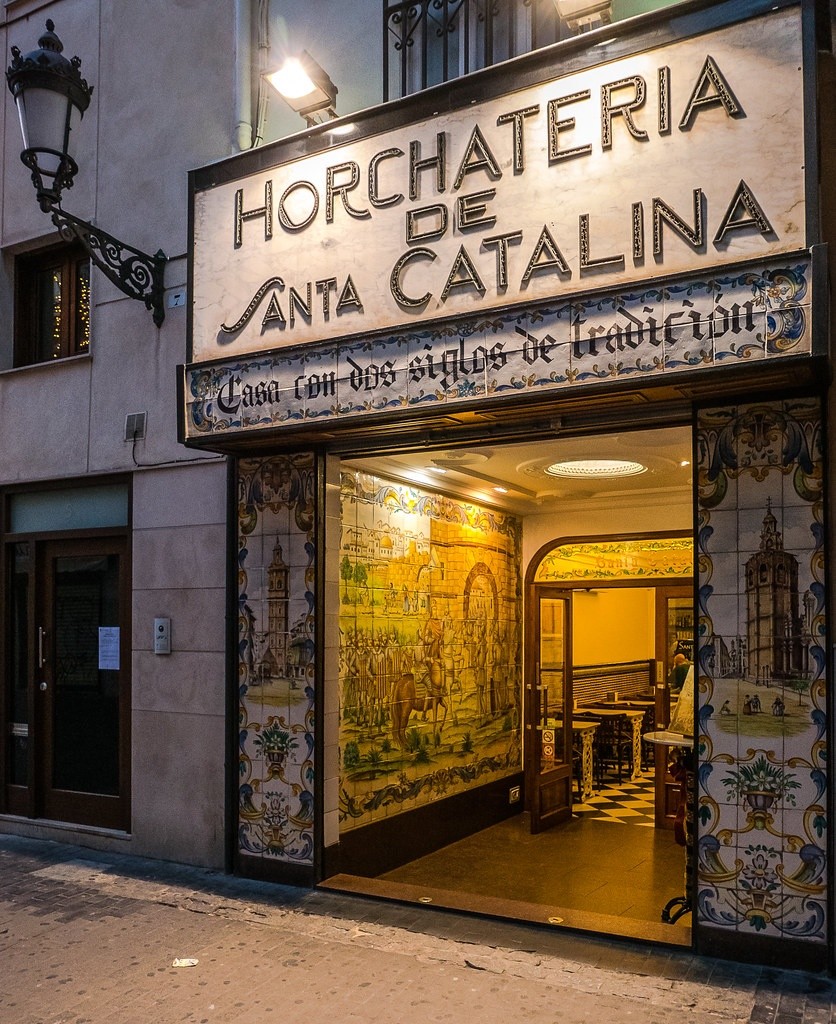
[556,694,655,802]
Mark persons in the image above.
[669,654,690,691]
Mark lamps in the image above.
[259,50,341,128]
[5,16,167,329]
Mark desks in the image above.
[637,693,680,698]
[599,701,677,724]
[556,719,600,803]
[553,708,646,780]
